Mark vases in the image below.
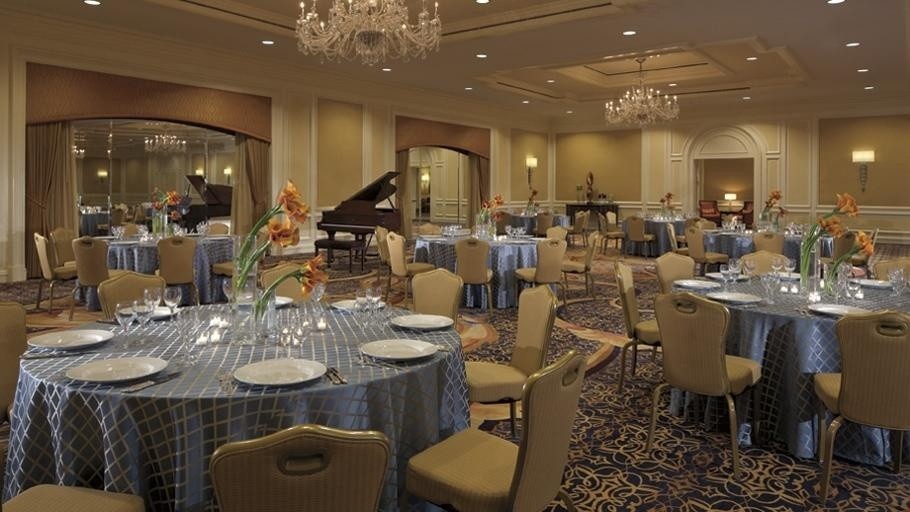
[772,222,779,231]
[825,262,851,300]
[228,290,252,347]
[165,221,176,236]
[760,211,770,231]
[670,213,674,220]
[152,214,164,233]
[799,248,821,296]
[255,300,278,347]
[666,206,671,219]
[661,208,665,219]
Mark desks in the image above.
[565,203,620,235]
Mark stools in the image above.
[315,241,365,272]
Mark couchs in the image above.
[699,199,721,225]
[737,201,755,229]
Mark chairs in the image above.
[496,212,511,235]
[667,223,690,257]
[98,272,166,319]
[657,252,695,292]
[742,251,789,280]
[34,233,77,312]
[133,207,150,227]
[0,303,27,424]
[571,210,590,246]
[615,262,663,392]
[515,240,566,310]
[51,228,80,268]
[677,219,695,249]
[411,268,464,319]
[418,223,441,236]
[752,231,785,255]
[374,227,415,286]
[207,224,227,238]
[97,208,124,238]
[69,237,130,319]
[385,232,434,308]
[212,235,258,302]
[464,284,557,438]
[211,424,391,512]
[533,213,553,236]
[607,211,624,251]
[814,309,910,504]
[567,212,586,249]
[0,484,145,512]
[546,226,568,239]
[402,350,587,512]
[158,236,200,307]
[685,227,728,276]
[562,231,598,300]
[646,292,760,481]
[822,228,879,280]
[623,217,657,260]
[875,257,910,282]
[599,213,625,256]
[699,219,717,231]
[454,238,492,316]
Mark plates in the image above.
[64,356,168,383]
[362,339,438,360]
[768,271,807,280]
[673,279,723,291]
[705,291,763,305]
[392,315,454,328]
[229,295,295,307]
[808,303,870,318]
[233,357,328,386]
[26,329,115,352]
[849,278,892,288]
[706,271,750,282]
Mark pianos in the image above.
[316,171,404,260]
[176,174,232,233]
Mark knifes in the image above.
[330,367,348,383]
[19,350,83,361]
[120,370,183,393]
[325,363,341,384]
[96,319,119,325]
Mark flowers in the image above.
[152,201,162,231]
[240,182,308,263]
[826,232,870,296]
[669,207,673,219]
[660,198,665,215]
[256,251,325,319]
[159,189,181,230]
[238,220,300,291]
[666,193,672,215]
[773,208,789,232]
[799,192,855,288]
[167,211,178,234]
[474,192,506,225]
[761,191,782,229]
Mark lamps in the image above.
[725,193,736,210]
[604,58,679,130]
[852,151,877,193]
[295,0,440,69]
[144,124,183,155]
[526,158,537,167]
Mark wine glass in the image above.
[132,296,155,348]
[505,225,526,241]
[768,256,784,274]
[766,273,781,300]
[351,310,391,339]
[761,273,779,305]
[719,264,732,291]
[310,281,326,301]
[163,286,182,327]
[181,303,326,366]
[783,258,797,277]
[743,259,756,285]
[440,224,462,240]
[727,257,743,286]
[114,300,138,349]
[222,277,235,305]
[142,287,162,328]
[355,290,370,309]
[330,297,387,311]
[888,267,906,298]
[365,285,382,306]
[846,278,862,306]
